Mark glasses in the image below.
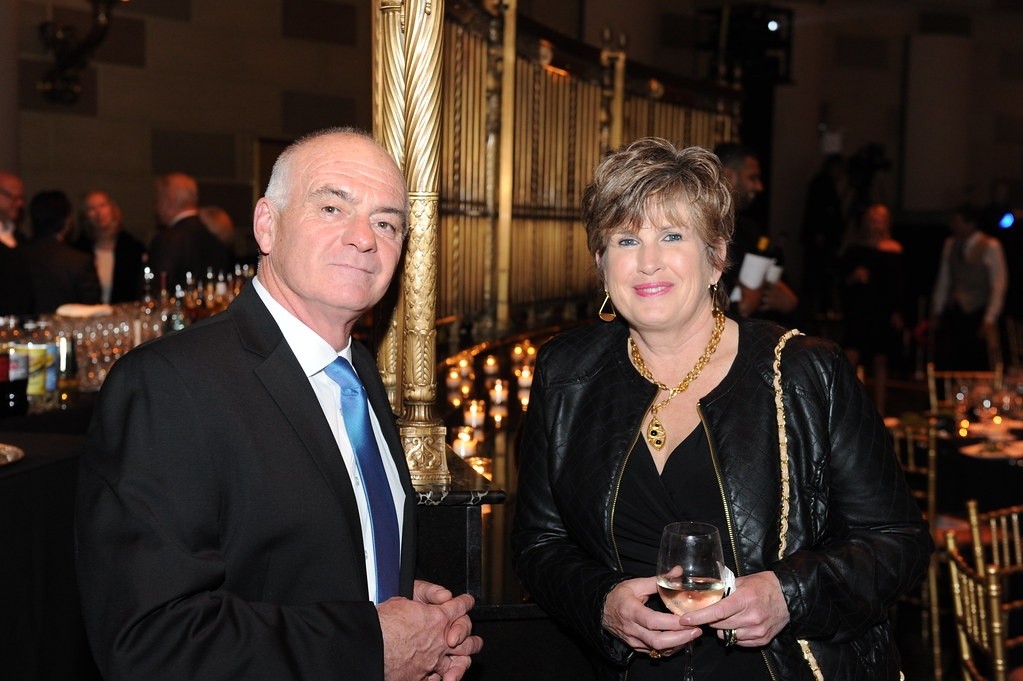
[0,187,27,201]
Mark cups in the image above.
[444,334,556,514]
[953,380,1022,451]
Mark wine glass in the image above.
[654,523,726,681]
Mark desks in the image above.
[887,402,1023,536]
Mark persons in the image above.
[0,169,231,315]
[74,127,483,681]
[516,137,936,681]
[713,141,1007,373]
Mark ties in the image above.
[324,356,402,608]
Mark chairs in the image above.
[927,362,1003,410]
[891,412,1023,681]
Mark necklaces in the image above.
[629,307,725,451]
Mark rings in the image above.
[722,630,738,648]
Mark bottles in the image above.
[0,253,256,416]
